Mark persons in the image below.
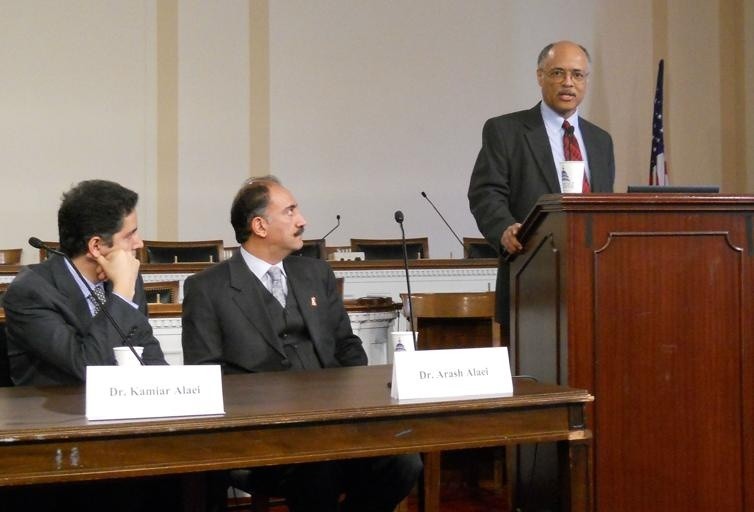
[468,41,615,360]
[0,178,170,387]
[180,175,423,512]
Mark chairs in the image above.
[0,249,22,265]
[137,240,224,262]
[336,277,345,295]
[401,293,518,512]
[292,239,327,259]
[350,238,429,259]
[463,237,499,258]
[40,241,61,262]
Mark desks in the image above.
[0,365,594,512]
[0,298,403,364]
[0,258,499,302]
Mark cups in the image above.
[559,160,586,194]
[388,330,419,354]
[112,346,145,366]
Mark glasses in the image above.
[540,67,592,83]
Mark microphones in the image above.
[421,191,473,258]
[29,237,154,377]
[318,212,341,252]
[394,210,418,352]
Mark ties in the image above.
[89,286,108,312]
[564,120,592,194]
[269,266,287,305]
